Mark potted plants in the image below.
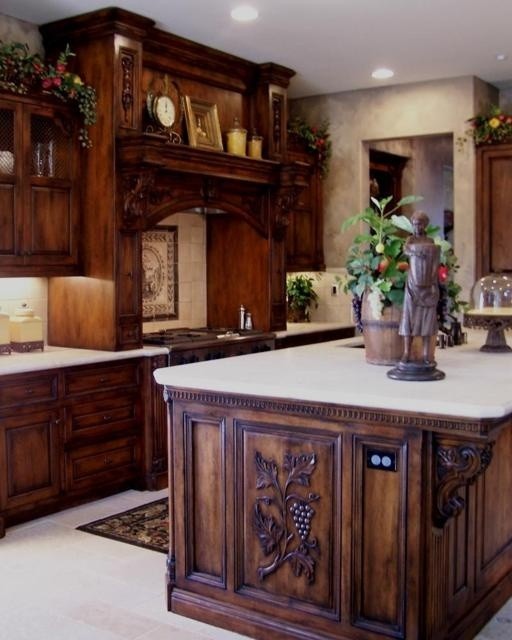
[286,271,321,325]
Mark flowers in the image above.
[452,100,510,152]
[0,38,98,150]
[347,191,464,331]
[289,114,331,180]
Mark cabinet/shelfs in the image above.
[0,370,63,539]
[280,131,325,268]
[0,91,80,275]
[63,358,144,503]
[476,144,511,281]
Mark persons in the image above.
[395,210,441,364]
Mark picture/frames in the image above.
[183,95,226,152]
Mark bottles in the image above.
[11,302,45,344]
[245,311,254,330]
[237,304,244,329]
[225,116,247,158]
[0,307,11,345]
[248,127,263,159]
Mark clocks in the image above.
[145,74,182,145]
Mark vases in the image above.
[356,275,407,366]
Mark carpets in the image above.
[74,493,169,554]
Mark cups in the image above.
[36,143,54,178]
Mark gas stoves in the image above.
[144,326,274,366]
